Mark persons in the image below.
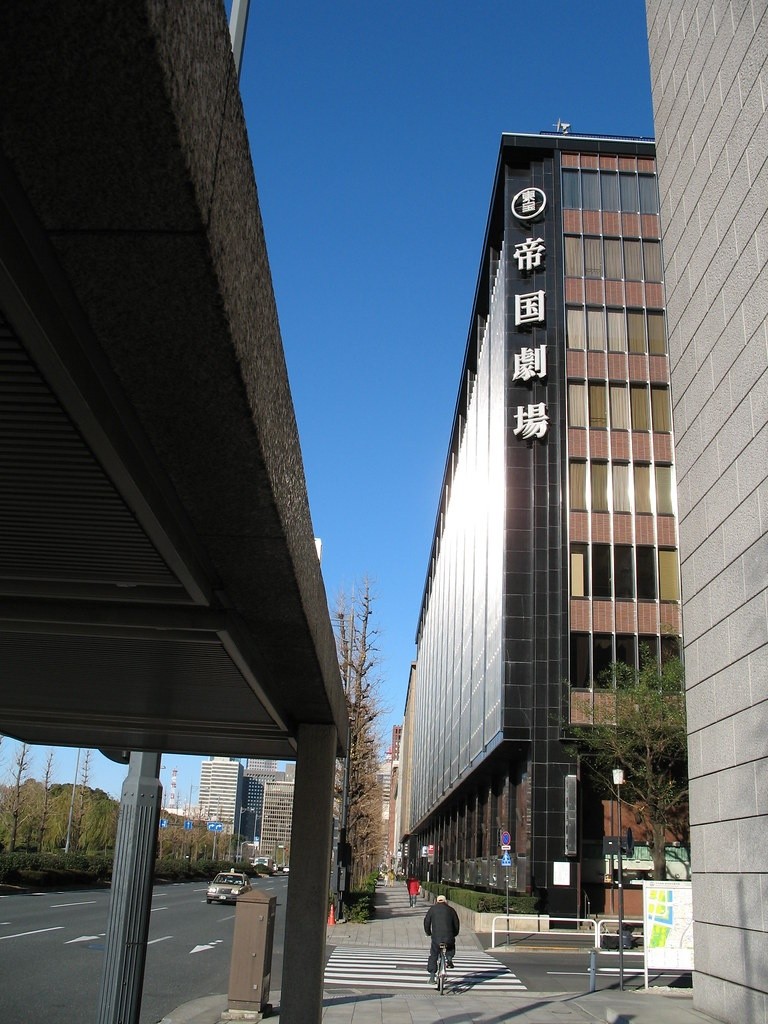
[384,873,389,887]
[407,874,420,908]
[388,869,395,888]
[424,895,460,985]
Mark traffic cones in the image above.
[328,903,336,925]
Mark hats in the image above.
[437,895,446,903]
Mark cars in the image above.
[283,866,289,873]
[273,864,279,872]
[206,867,251,905]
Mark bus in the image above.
[254,856,275,871]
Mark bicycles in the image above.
[425,933,451,995]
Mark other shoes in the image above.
[447,961,454,968]
[428,979,436,984]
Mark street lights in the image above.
[612,764,624,989]
[254,813,268,848]
[235,806,254,861]
